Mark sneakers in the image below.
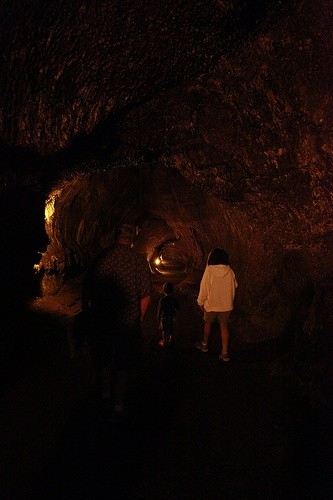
[217,350,229,361]
[194,340,208,353]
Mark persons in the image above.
[158,281,178,347]
[196,247,238,361]
